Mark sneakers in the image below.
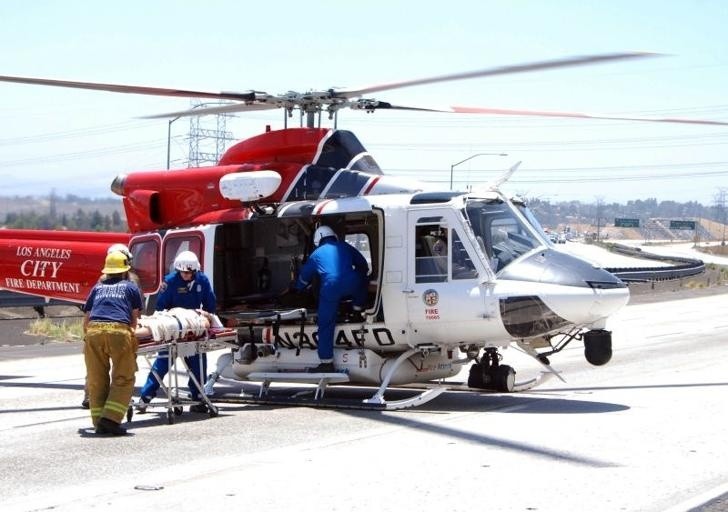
[81,398,90,408]
[95,417,128,437]
[307,361,335,373]
[189,404,208,413]
[137,394,151,411]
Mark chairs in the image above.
[422,235,445,282]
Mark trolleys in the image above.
[116,325,240,426]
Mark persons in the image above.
[82,245,142,409]
[293,224,370,372]
[81,253,143,439]
[134,251,217,413]
[431,229,477,280]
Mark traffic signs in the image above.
[668,220,697,230]
[615,218,640,228]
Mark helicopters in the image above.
[0,45,728,415]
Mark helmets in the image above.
[100,250,132,274]
[174,250,201,273]
[106,243,134,262]
[313,224,340,248]
[440,217,455,230]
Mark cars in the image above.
[545,229,609,244]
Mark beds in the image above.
[79,325,241,427]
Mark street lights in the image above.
[448,152,510,190]
[164,100,241,170]
